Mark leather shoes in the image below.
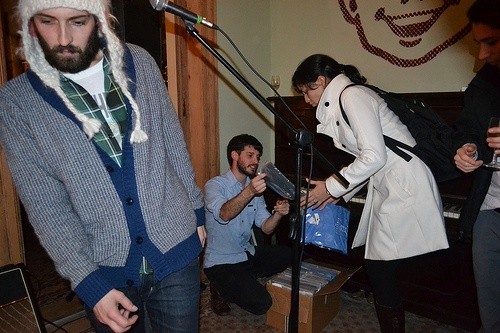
[210,282,231,316]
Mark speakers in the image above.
[0,263,48,333]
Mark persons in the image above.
[292,54,450,333]
[203,133,291,315]
[0,0,207,333]
[452,0,500,333]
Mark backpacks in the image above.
[339,84,456,176]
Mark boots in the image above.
[374,302,405,333]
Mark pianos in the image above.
[267,91,482,333]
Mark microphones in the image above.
[150,0,218,29]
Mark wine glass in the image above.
[482,117,500,170]
[271,76,281,98]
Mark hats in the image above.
[18,0,149,145]
